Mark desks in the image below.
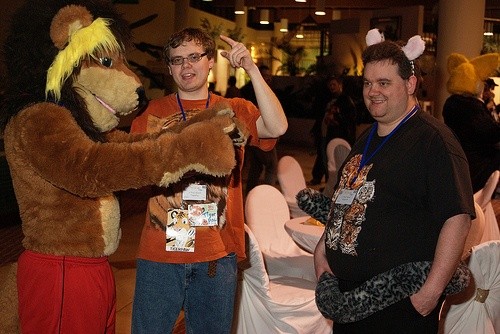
[284,217,325,253]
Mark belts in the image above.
[207,261,217,278]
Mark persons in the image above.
[312,41,476,334]
[442,53,500,245]
[208,82,222,96]
[237,66,278,196]
[224,76,240,99]
[481,78,500,125]
[310,76,361,185]
[128,28,288,334]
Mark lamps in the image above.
[260,9,269,24]
[234,0,244,15]
[280,18,288,32]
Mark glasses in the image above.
[172,52,208,65]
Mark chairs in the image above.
[234,136,500,334]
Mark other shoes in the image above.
[306,178,321,186]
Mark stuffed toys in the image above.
[4,6,252,334]
